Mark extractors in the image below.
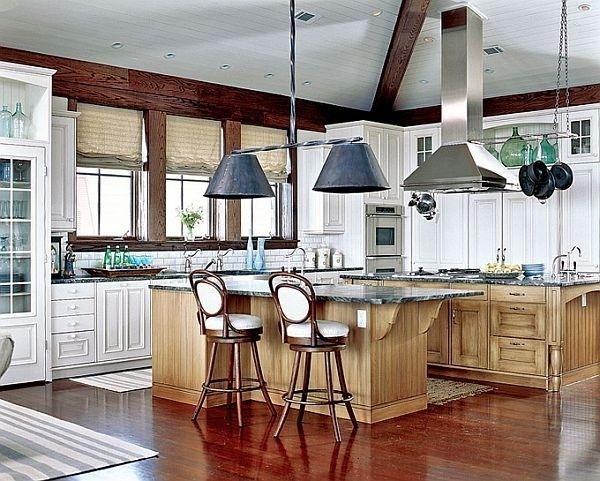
[401,7,522,193]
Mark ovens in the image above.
[366,202,402,255]
[366,255,403,273]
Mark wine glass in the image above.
[128,254,155,269]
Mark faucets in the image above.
[185,249,202,273]
[205,260,216,271]
[218,248,234,269]
[567,246,582,271]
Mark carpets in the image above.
[70,366,152,393]
[425,378,498,405]
[0,395,159,481]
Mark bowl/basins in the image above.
[576,146,590,153]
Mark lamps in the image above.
[203,0,392,200]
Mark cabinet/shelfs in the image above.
[51,283,95,379]
[50,95,82,234]
[414,283,547,394]
[0,61,56,385]
[96,281,151,374]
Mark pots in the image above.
[406,191,439,219]
[516,159,573,205]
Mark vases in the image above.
[187,226,195,241]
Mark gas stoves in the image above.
[390,265,485,278]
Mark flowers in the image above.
[175,203,204,228]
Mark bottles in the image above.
[304,240,343,268]
[123,244,131,268]
[531,132,556,162]
[500,124,528,166]
[0,105,15,137]
[103,245,112,269]
[553,138,558,161]
[113,245,123,268]
[520,143,533,165]
[485,143,499,159]
[11,101,31,138]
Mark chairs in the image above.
[267,273,358,444]
[189,269,278,428]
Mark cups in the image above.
[0,157,29,274]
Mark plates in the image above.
[0,273,26,294]
[478,269,524,278]
[522,262,545,276]
